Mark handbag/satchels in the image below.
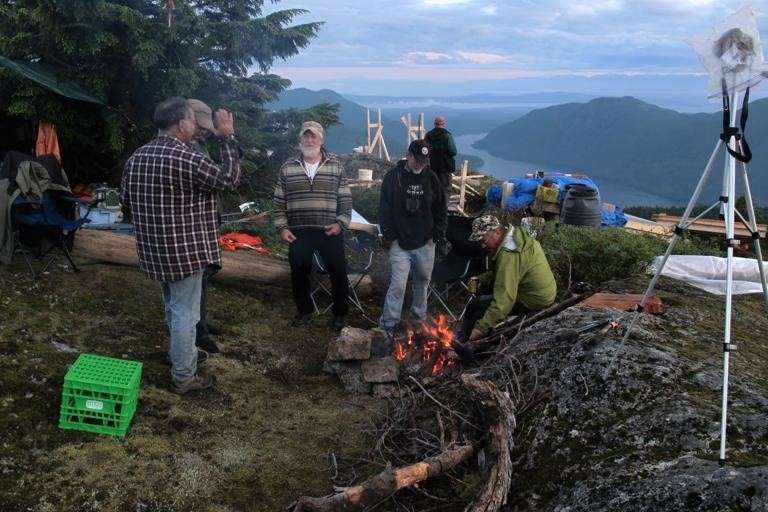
[468,190,485,211]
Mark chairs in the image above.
[1,151,92,279]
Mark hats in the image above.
[410,139,432,167]
[299,121,324,137]
[467,215,500,242]
[188,99,216,136]
[436,116,445,124]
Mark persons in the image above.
[183,97,225,354]
[271,120,353,331]
[377,139,450,339]
[118,95,244,394]
[453,213,557,353]
[423,115,457,209]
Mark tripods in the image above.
[602,70,767,468]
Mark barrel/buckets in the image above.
[359,169,373,180]
[559,183,586,216]
[559,186,600,231]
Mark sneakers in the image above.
[166,323,220,394]
[292,312,314,326]
[333,316,345,331]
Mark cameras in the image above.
[713,30,754,69]
